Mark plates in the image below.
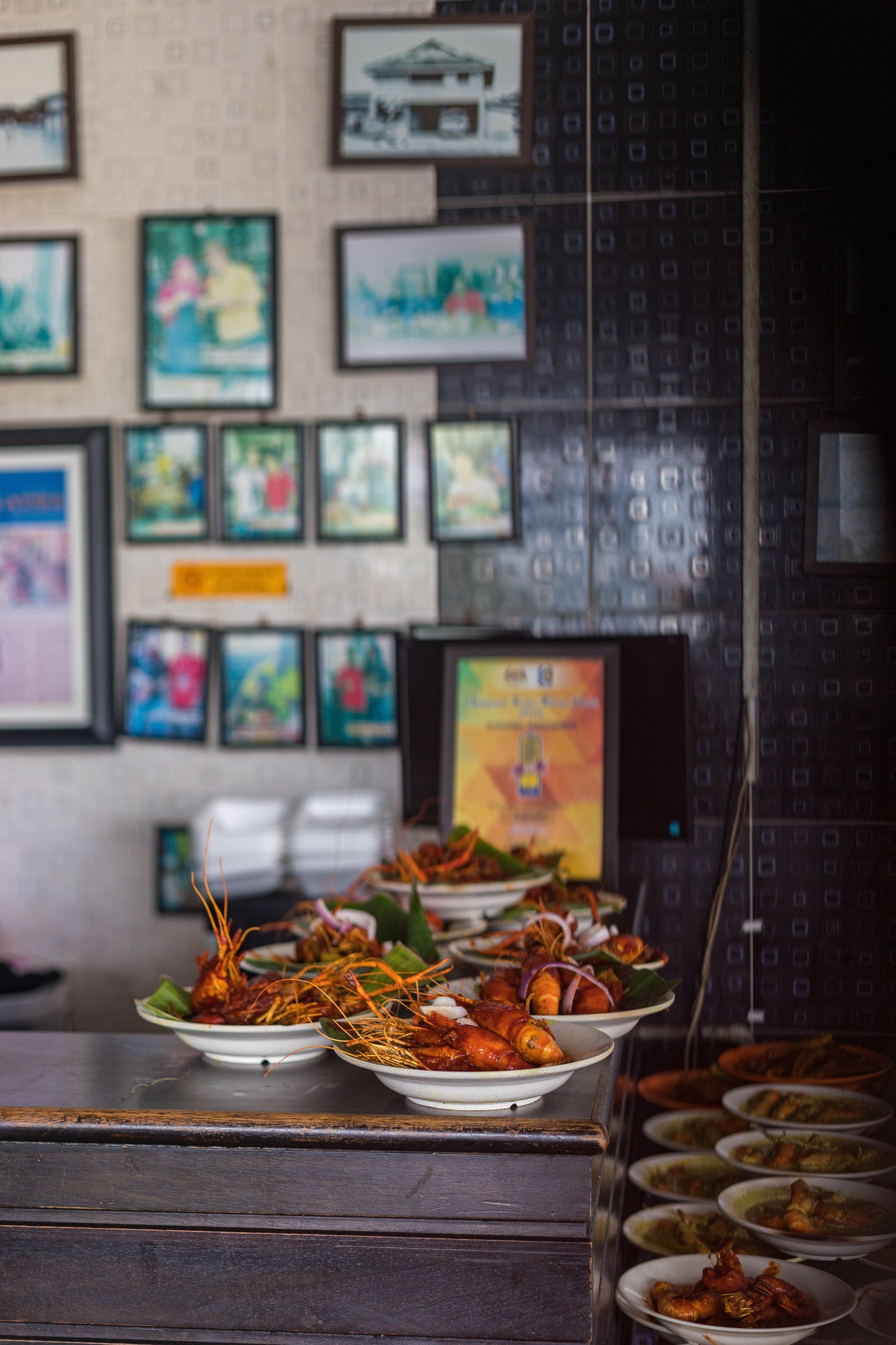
[332,1017,614,1111]
[614,1039,896,1345]
[426,976,675,1040]
[367,864,552,918]
[136,985,391,1064]
[235,888,669,976]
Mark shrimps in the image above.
[624,1025,895,1345]
[178,798,651,1076]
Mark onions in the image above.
[523,912,572,947]
[573,923,618,954]
[315,898,351,934]
[310,908,377,941]
[561,964,595,1014]
[518,962,614,1007]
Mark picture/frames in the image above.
[331,12,536,168]
[424,414,519,546]
[0,32,78,181]
[218,623,308,746]
[441,640,624,894]
[801,416,896,577]
[312,626,401,749]
[1,229,81,379]
[135,211,282,412]
[1,424,115,749]
[312,416,406,544]
[218,421,305,542]
[121,420,214,545]
[118,618,215,750]
[333,215,538,371]
[156,822,204,913]
